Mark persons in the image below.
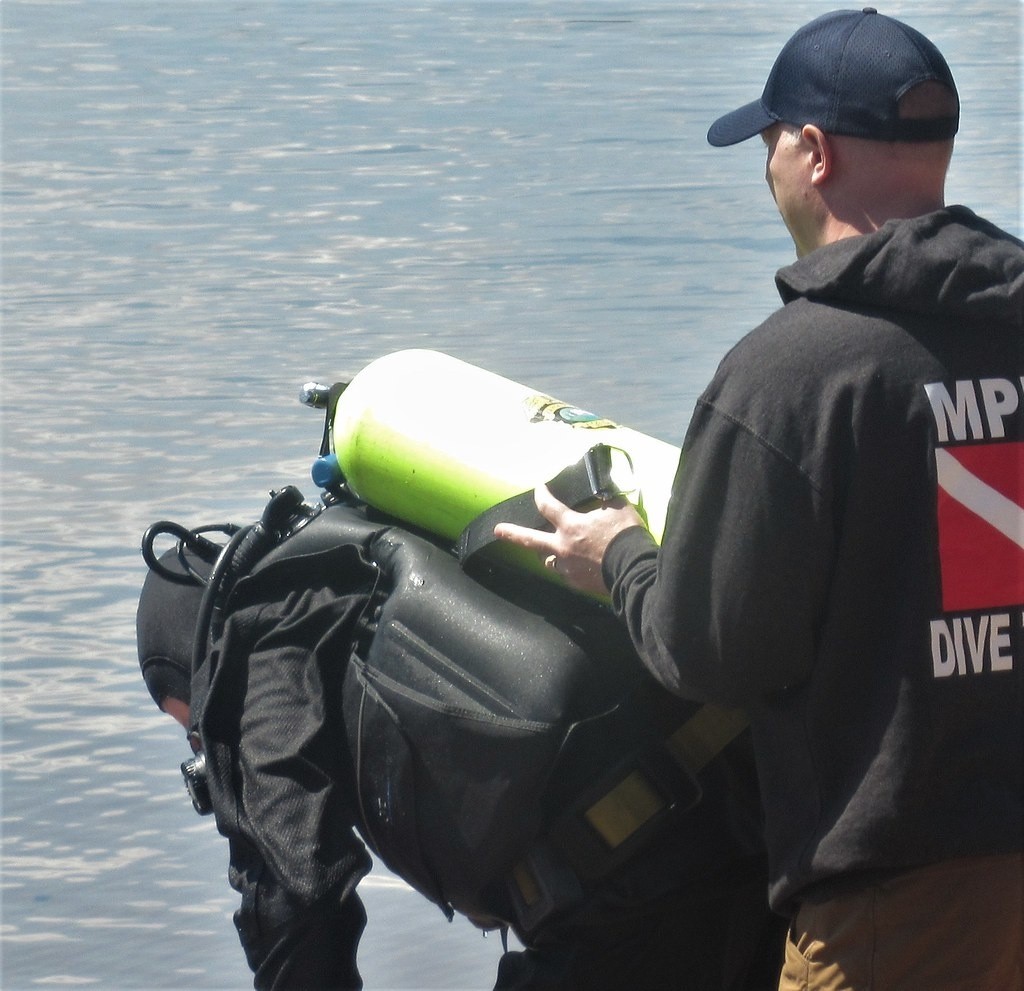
[138,503,791,991]
[492,6,1024,991]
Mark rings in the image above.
[550,556,561,576]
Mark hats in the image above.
[706,8,960,148]
